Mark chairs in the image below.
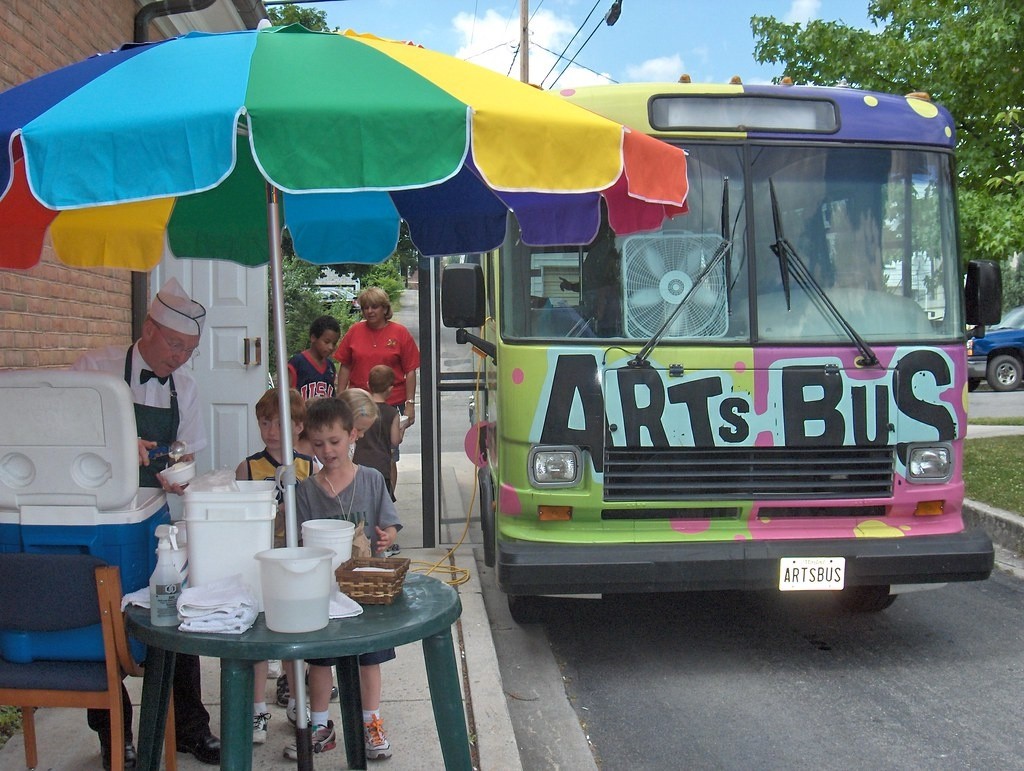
[1,554,177,771]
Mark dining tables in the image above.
[122,591,474,771]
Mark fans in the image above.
[621,232,728,339]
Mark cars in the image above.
[966,302,1024,394]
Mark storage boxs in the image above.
[0,368,170,662]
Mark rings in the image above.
[410,420,412,423]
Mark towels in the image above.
[121,572,364,636]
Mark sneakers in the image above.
[286,703,311,729]
[363,713,393,761]
[283,720,336,760]
[305,669,338,700]
[253,713,271,743]
[277,674,290,707]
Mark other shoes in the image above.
[267,662,280,678]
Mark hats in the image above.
[150,277,206,336]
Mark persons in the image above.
[560,216,618,338]
[282,397,404,760]
[333,286,424,491]
[73,275,221,771]
[287,314,341,404]
[235,365,422,707]
[234,388,316,745]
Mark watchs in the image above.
[405,399,414,405]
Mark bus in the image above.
[440,75,1002,627]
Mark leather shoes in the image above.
[101,743,136,771]
[175,730,221,766]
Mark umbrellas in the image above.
[0,19,691,771]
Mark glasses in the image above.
[153,321,201,359]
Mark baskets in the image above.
[335,557,410,605]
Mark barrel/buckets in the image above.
[300,518,356,570]
[253,546,337,633]
[184,480,277,613]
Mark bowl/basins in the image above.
[398,415,408,429]
[158,460,196,486]
